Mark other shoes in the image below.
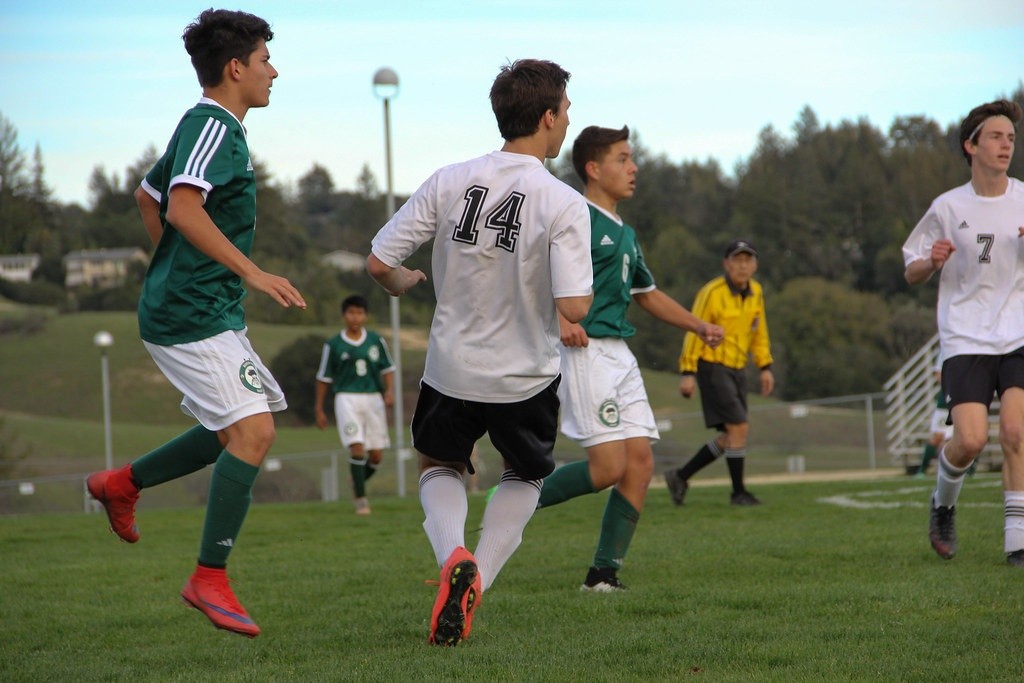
[730,493,761,506]
[664,469,688,506]
[354,505,370,515]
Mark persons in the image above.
[902,99,1024,565]
[365,57,594,647]
[314,294,396,516]
[484,124,726,592]
[664,240,775,509]
[88,6,308,639]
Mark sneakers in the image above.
[86,462,141,544]
[579,566,630,593]
[1007,550,1024,564]
[929,490,957,559]
[431,546,478,646]
[428,573,482,644]
[180,565,261,638]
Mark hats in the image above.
[724,241,757,258]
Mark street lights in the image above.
[374,65,404,499]
[93,330,114,471]
